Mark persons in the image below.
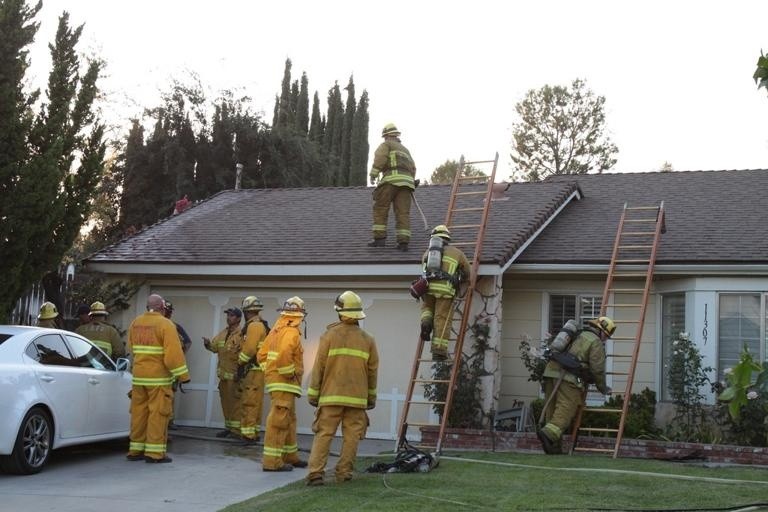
[420,224,470,360]
[74,301,127,369]
[125,293,191,464]
[232,295,272,446]
[536,316,617,454]
[256,296,309,471]
[66,303,92,332]
[305,290,379,486]
[35,302,60,328]
[201,307,242,438]
[165,300,192,431]
[367,123,415,248]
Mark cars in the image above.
[0,320,132,477]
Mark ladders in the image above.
[568,200,665,459]
[394,152,501,454]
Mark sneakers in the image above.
[368,239,384,246]
[396,243,409,251]
[263,464,292,471]
[168,421,178,429]
[292,460,308,467]
[421,319,430,341]
[433,353,448,360]
[307,478,323,485]
[146,456,172,462]
[537,429,560,454]
[216,430,260,446]
[127,455,145,461]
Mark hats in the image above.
[74,305,90,318]
[165,299,173,310]
[224,308,241,316]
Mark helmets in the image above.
[431,225,450,239]
[334,291,365,319]
[38,302,59,319]
[279,296,305,316]
[588,317,616,339]
[88,301,109,316]
[382,124,401,136]
[243,296,264,311]
